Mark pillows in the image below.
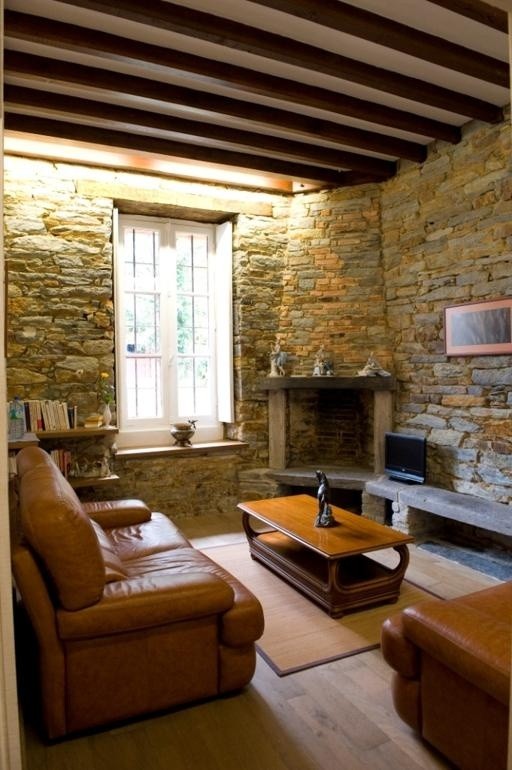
[15,444,133,612]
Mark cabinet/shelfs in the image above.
[7,423,120,495]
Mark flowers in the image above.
[97,373,116,404]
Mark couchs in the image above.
[10,446,265,745]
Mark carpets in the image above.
[189,539,450,676]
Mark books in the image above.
[7,399,78,432]
[9,450,73,484]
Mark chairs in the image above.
[378,576,512,769]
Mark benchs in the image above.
[365,478,512,543]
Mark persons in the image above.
[312,344,334,375]
[267,338,286,376]
[362,352,391,376]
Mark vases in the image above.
[103,404,112,428]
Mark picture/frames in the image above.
[443,298,512,356]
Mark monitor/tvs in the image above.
[384,433,427,485]
